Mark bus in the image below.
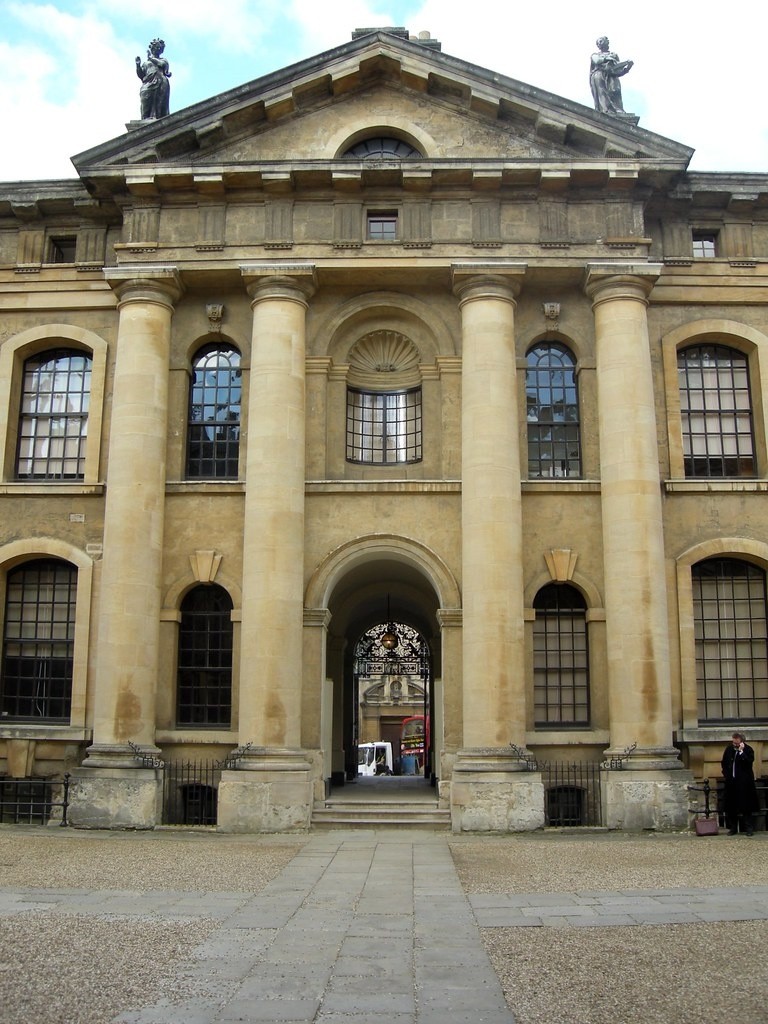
[400,715,430,767]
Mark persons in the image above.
[135,38,170,119]
[375,726,423,776]
[721,733,761,836]
[590,36,633,114]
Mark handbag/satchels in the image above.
[694,817,718,835]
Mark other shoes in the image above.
[746,829,754,836]
[727,828,739,836]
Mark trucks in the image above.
[358,742,394,777]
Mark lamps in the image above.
[381,593,397,648]
